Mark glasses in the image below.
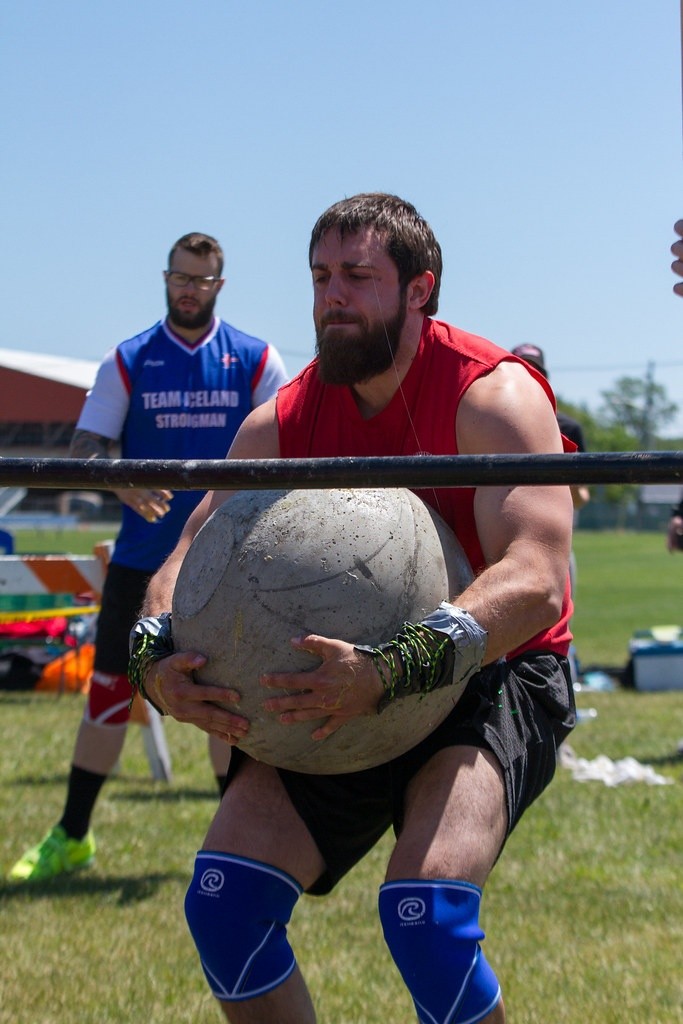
[166,270,219,291]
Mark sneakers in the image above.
[6,823,96,882]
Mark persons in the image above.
[128,189,584,1011]
[505,342,585,685]
[4,230,292,891]
